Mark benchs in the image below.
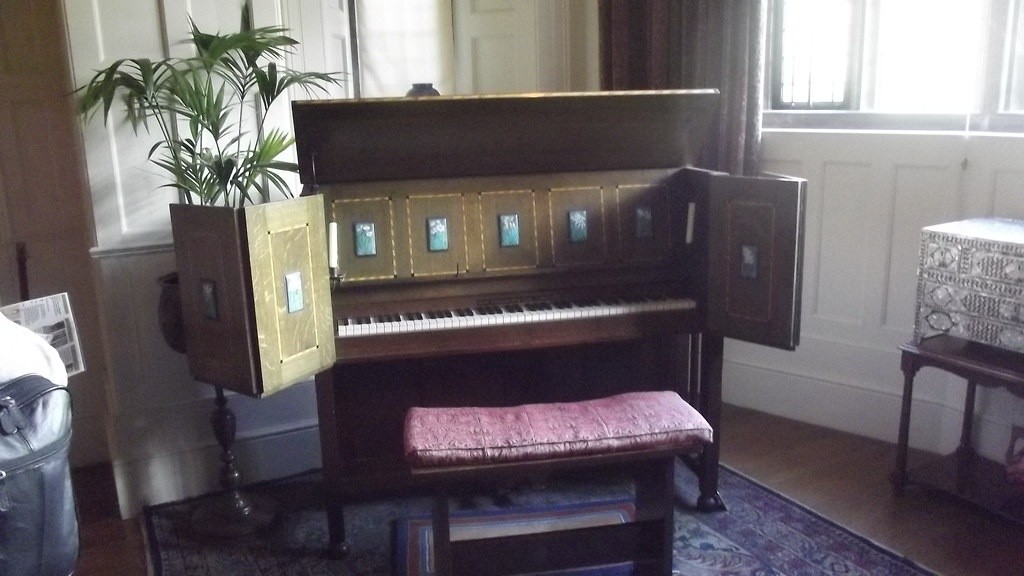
[360,389,713,576]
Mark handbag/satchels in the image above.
[0,375,80,576]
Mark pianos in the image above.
[175,90,812,554]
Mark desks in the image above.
[888,332,1023,532]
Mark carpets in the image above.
[140,458,936,575]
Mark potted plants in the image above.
[64,5,346,541]
